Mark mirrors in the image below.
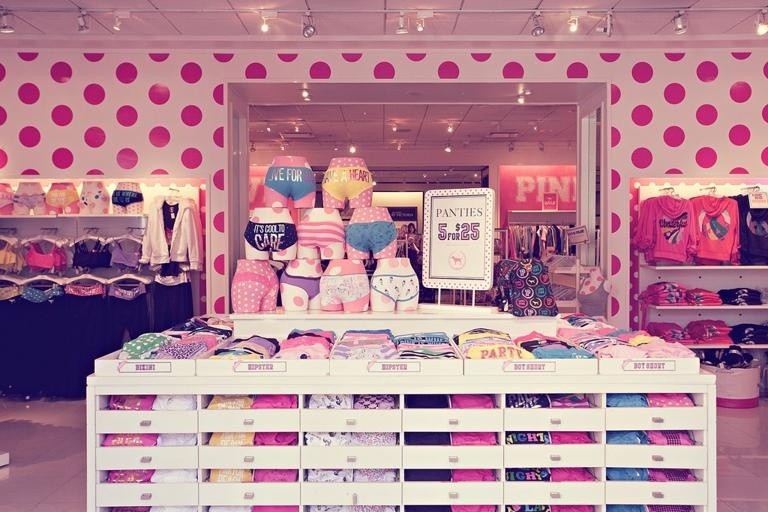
[309,163,491,305]
[242,99,585,312]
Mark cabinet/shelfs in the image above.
[459,226,511,306]
[632,260,767,356]
[80,325,720,512]
[536,252,582,314]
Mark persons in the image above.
[231,155,420,313]
[0,180,145,217]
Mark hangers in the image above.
[656,183,767,200]
[506,221,577,234]
[1,188,198,296]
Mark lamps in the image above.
[0,2,767,43]
